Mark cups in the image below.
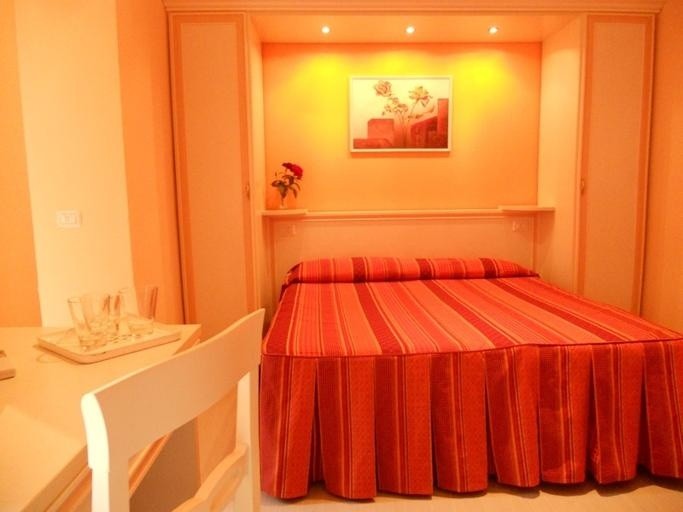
[65,291,112,351]
[118,284,159,337]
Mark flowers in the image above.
[373,81,436,129]
[271,162,303,199]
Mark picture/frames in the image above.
[351,77,452,152]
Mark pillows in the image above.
[289,256,539,283]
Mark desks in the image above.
[0,323,201,512]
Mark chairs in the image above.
[81,308,265,512]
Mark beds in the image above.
[259,258,683,501]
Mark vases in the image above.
[278,194,288,209]
